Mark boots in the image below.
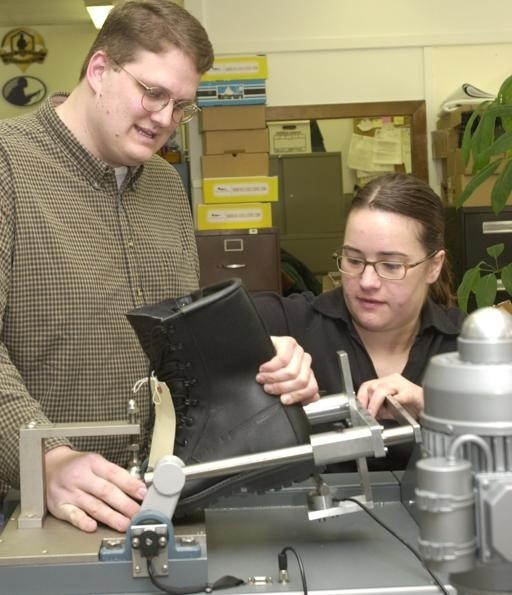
[123,278,326,518]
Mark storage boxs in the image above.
[431,104,512,209]
[196,53,279,232]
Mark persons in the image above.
[250,172,471,472]
[0,0,217,535]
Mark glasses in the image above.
[115,60,202,126]
[330,250,438,281]
